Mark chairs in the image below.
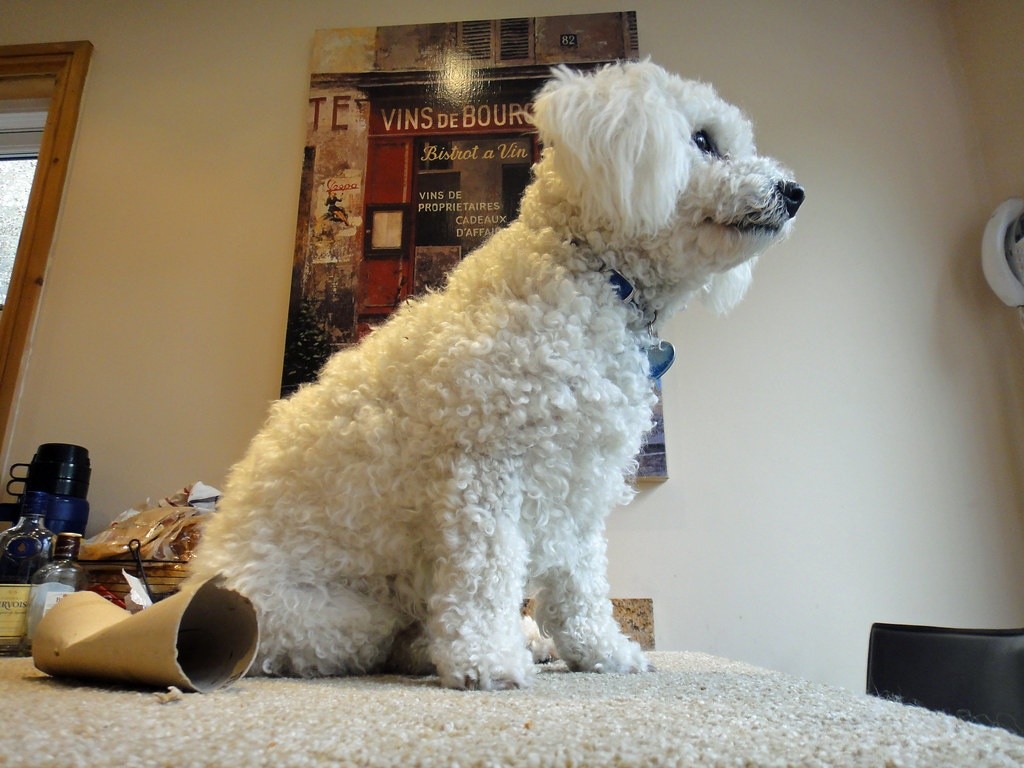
[866,623,1024,738]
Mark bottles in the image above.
[18,532,89,657]
[0,491,57,656]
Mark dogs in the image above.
[179,59,804,692]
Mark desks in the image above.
[0,649,1024,768]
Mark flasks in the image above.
[0,442,91,537]
